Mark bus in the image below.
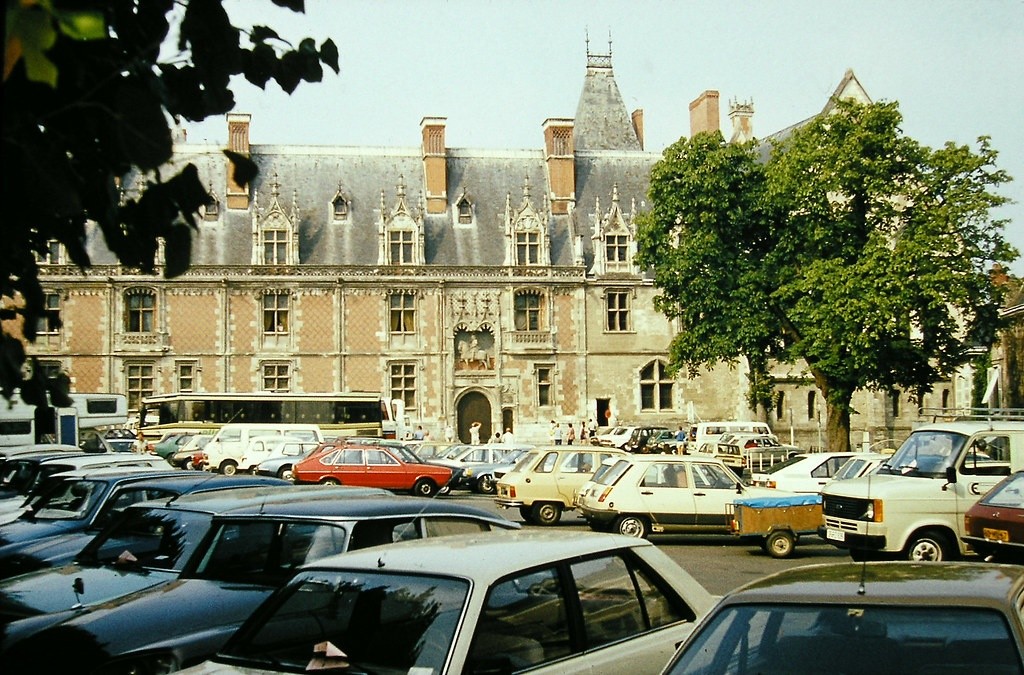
[135,389,383,455]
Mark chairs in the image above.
[663,468,688,488]
[579,461,592,471]
[521,595,609,660]
[179,414,350,424]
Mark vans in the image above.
[817,406,1024,563]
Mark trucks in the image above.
[0,391,128,448]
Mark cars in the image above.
[656,561,1024,675]
[0,496,554,675]
[832,446,896,480]
[588,422,807,478]
[0,422,580,585]
[291,444,453,498]
[492,446,664,527]
[168,528,771,675]
[960,469,1024,566]
[571,455,800,540]
[750,451,876,496]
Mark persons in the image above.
[468,422,482,446]
[501,428,515,445]
[414,425,425,440]
[550,420,564,445]
[971,439,990,458]
[674,425,685,455]
[588,419,596,437]
[579,421,588,445]
[444,426,455,442]
[492,432,502,443]
[566,423,576,445]
[130,432,148,451]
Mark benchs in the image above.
[467,633,546,665]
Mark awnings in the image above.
[981,371,998,404]
